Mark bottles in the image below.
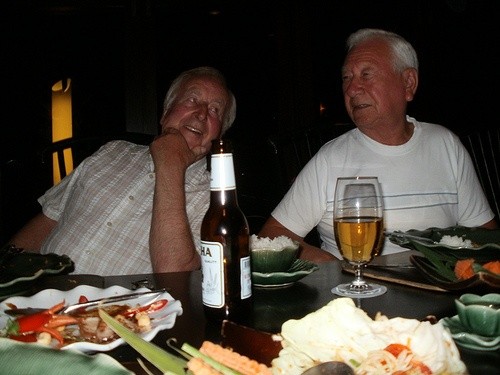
[200,139,255,327]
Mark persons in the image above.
[256,29,497,262]
[0,67,237,277]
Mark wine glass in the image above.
[332,177,385,298]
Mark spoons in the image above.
[4,288,170,316]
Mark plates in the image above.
[410,255,500,291]
[249,259,318,288]
[0,338,135,375]
[0,285,182,349]
[437,315,500,351]
[0,242,74,286]
[388,226,500,281]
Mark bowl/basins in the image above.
[453,293,500,337]
[250,240,302,272]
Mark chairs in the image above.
[268,118,356,248]
[424,117,500,224]
[0,133,153,249]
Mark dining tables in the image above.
[103,260,500,375]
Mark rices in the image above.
[250,234,298,250]
[433,235,474,248]
